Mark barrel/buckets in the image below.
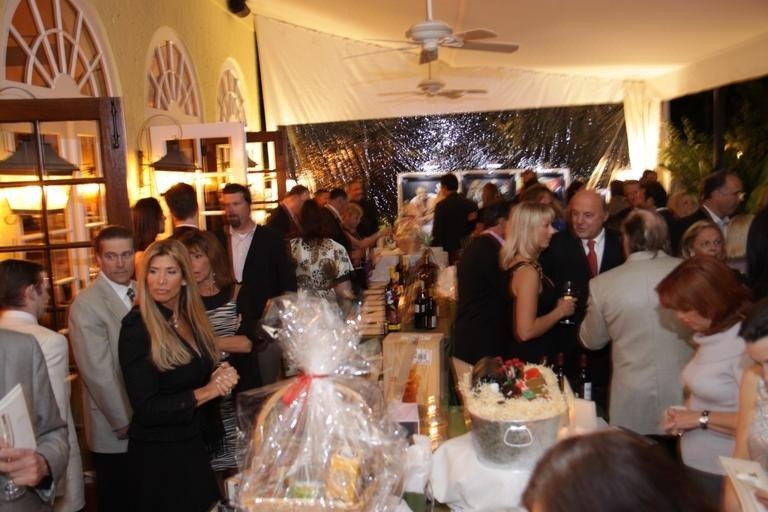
[464,403,559,471]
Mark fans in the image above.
[375,63,488,100]
[342,0,521,63]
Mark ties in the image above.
[128,289,136,303]
[586,240,598,276]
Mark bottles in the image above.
[556,351,569,394]
[495,354,550,400]
[382,252,441,332]
[575,354,593,401]
[559,279,575,325]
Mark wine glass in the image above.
[0,410,27,501]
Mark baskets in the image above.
[241,380,378,511]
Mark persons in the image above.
[724,297,768,512]
[179,229,258,482]
[654,253,752,512]
[68,224,139,512]
[520,428,718,512]
[0,167,768,456]
[0,258,87,512]
[0,328,71,512]
[119,238,241,512]
